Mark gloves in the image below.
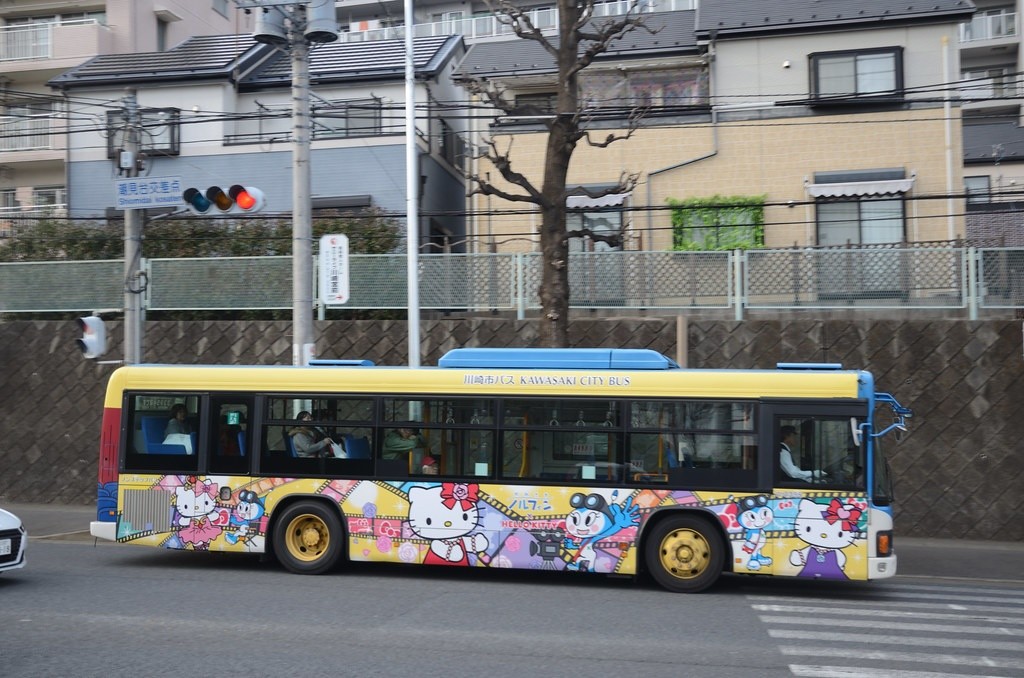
[814,470,828,478]
[814,479,827,483]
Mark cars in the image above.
[0,507,28,575]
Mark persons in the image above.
[161,403,192,454]
[382,425,428,460]
[287,410,332,459]
[778,424,829,486]
[316,412,349,445]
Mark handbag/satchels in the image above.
[328,438,346,458]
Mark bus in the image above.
[90,345,913,596]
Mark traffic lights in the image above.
[76,315,102,361]
[183,184,266,215]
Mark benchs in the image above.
[143,413,202,457]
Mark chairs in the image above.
[238,427,372,460]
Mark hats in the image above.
[421,455,436,466]
